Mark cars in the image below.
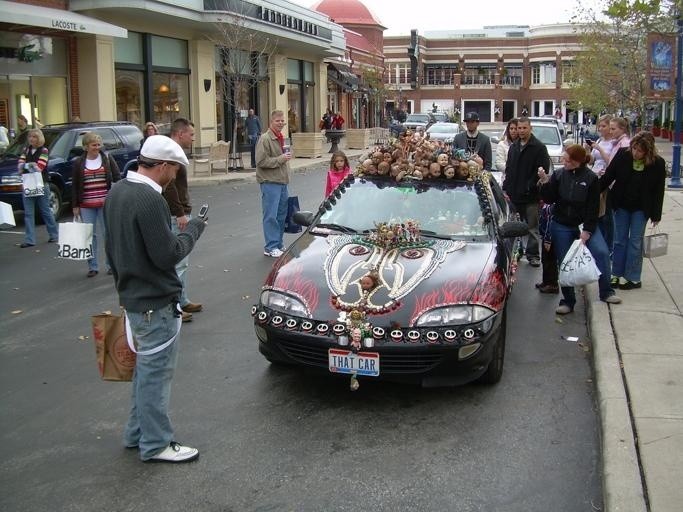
[251,163,530,390]
[395,108,465,151]
[491,116,572,171]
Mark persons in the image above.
[136,122,159,157]
[455,112,493,173]
[324,149,352,199]
[16,129,58,247]
[321,107,332,143]
[359,275,376,291]
[68,132,122,278]
[357,127,483,184]
[329,111,344,130]
[0,125,9,154]
[102,135,207,464]
[239,107,262,169]
[496,109,665,316]
[253,110,293,257]
[14,114,30,141]
[161,117,203,323]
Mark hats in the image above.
[140,135,189,166]
[463,112,480,121]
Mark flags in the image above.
[644,31,676,101]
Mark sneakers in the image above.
[147,441,199,464]
[264,248,282,257]
[529,258,541,267]
[601,275,641,302]
[535,281,559,294]
[87,270,96,277]
[21,243,30,247]
[555,305,571,314]
[182,303,201,321]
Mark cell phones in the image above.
[199,203,208,222]
[586,139,592,147]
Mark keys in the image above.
[142,308,153,324]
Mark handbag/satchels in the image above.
[91,313,136,381]
[21,172,45,197]
[556,224,601,287]
[58,221,94,260]
[284,195,302,233]
[643,226,668,258]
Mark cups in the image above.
[282,145,290,153]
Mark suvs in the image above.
[0,121,146,222]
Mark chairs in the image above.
[189,139,230,176]
